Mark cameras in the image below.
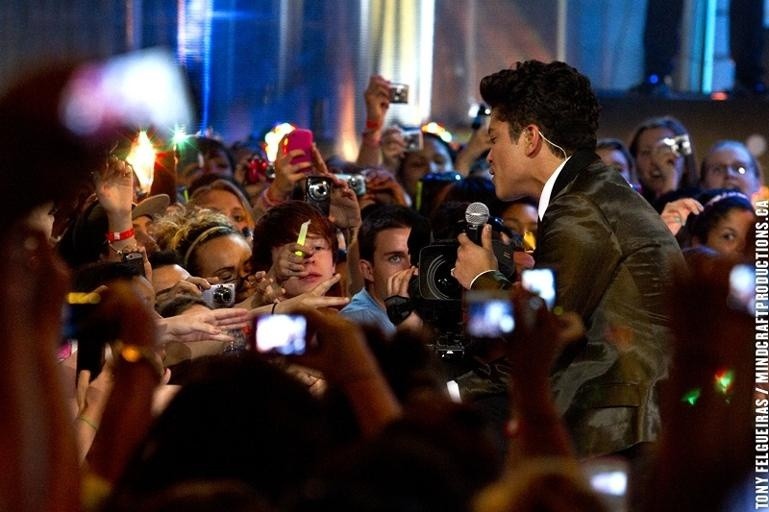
[338,172,366,198]
[304,176,331,217]
[389,83,411,104]
[674,132,694,155]
[200,282,236,307]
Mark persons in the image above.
[0,58,769,512]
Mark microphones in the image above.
[464,202,489,246]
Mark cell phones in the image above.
[286,129,314,170]
[123,254,150,280]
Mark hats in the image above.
[82,193,172,244]
